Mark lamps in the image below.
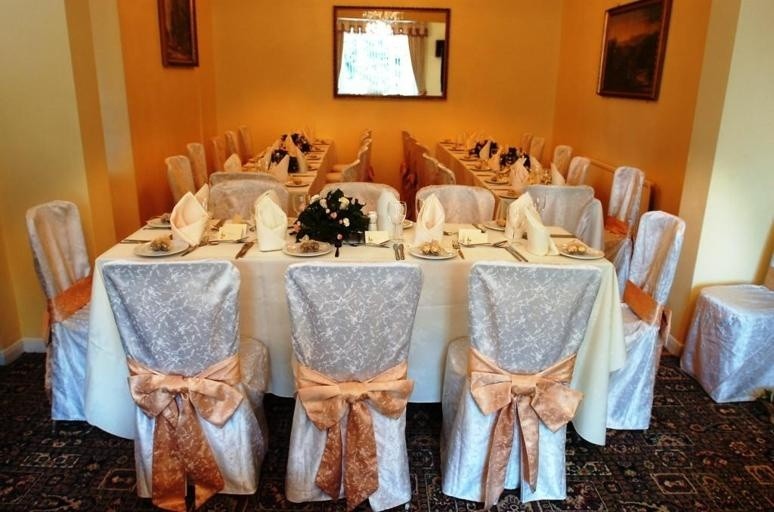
[361,9,406,25]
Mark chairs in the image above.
[401,130,603,250]
[606,210,686,430]
[284,262,425,512]
[165,124,371,218]
[441,262,602,511]
[25,201,93,421]
[605,166,645,299]
[318,182,402,235]
[679,243,774,407]
[97,258,270,512]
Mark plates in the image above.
[283,241,333,257]
[408,246,458,260]
[559,248,605,260]
[439,138,523,200]
[134,239,189,258]
[285,139,329,186]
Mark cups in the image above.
[528,193,547,218]
[256,158,268,172]
[290,193,311,215]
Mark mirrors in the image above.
[330,5,451,102]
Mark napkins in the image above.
[414,193,446,245]
[376,189,404,233]
[525,204,561,255]
[505,191,532,241]
[254,190,290,251]
[170,191,209,246]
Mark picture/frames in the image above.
[595,0,674,102]
[155,0,199,68]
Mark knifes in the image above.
[393,243,405,261]
[234,242,255,260]
[182,244,205,257]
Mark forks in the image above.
[452,239,465,260]
[494,241,529,262]
[333,242,345,257]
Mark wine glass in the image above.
[196,198,217,244]
[386,200,408,247]
